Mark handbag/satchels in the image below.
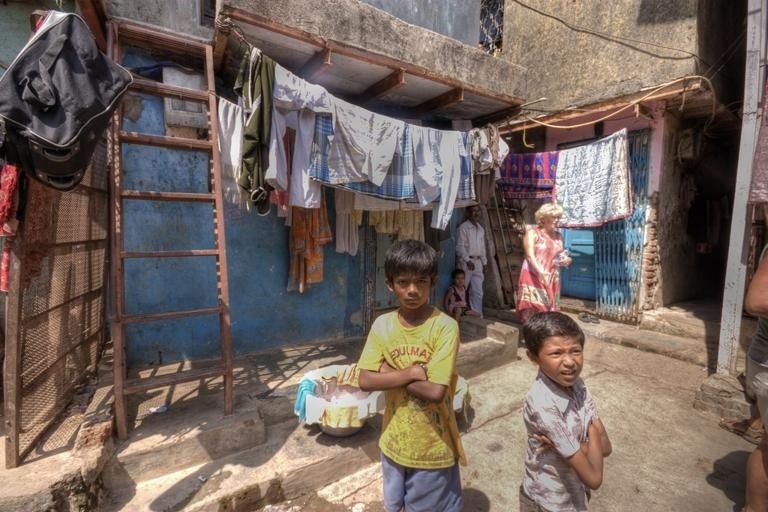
[1,6,134,194]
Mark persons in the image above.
[356,239,462,510]
[740,244,768,510]
[444,269,481,321]
[456,203,488,316]
[516,203,572,324]
[520,312,612,512]
